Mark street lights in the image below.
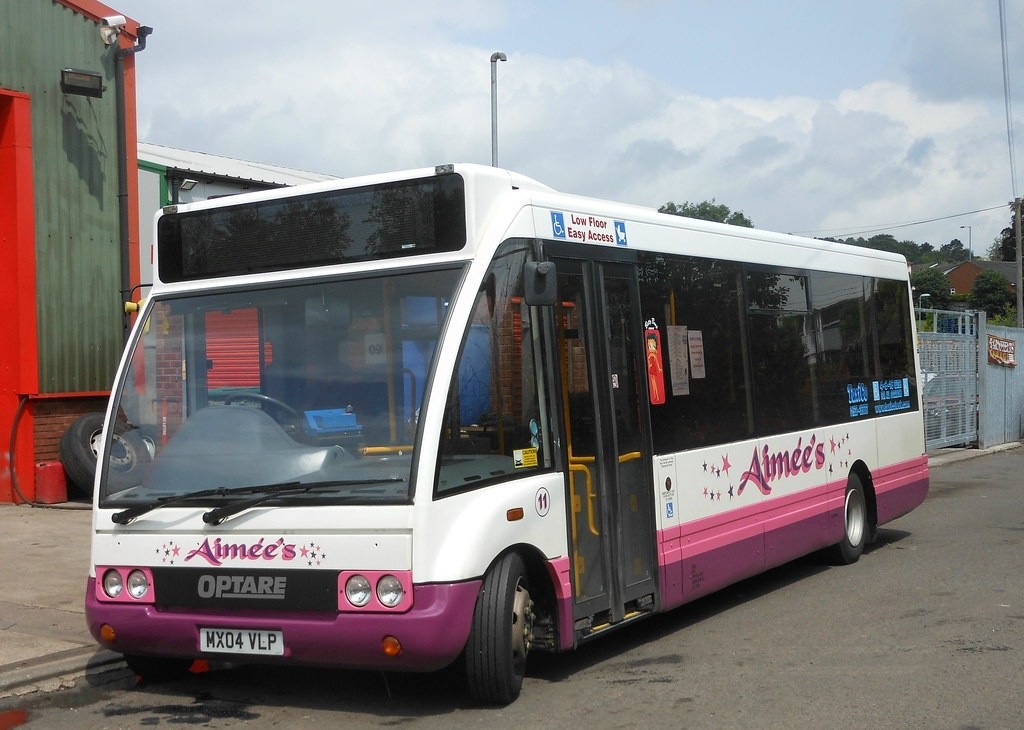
[959,225,972,261]
[919,293,931,332]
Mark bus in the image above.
[85,161,931,704]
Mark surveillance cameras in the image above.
[101,15,127,28]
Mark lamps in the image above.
[179,177,198,191]
[60,67,108,99]
